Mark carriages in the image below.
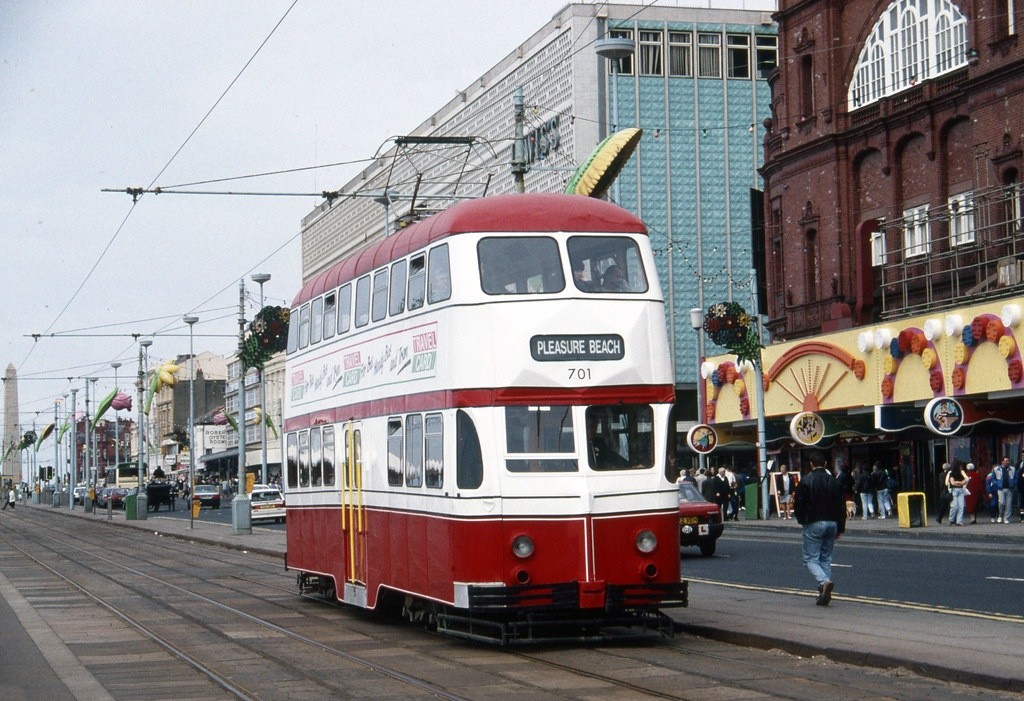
[145,481,177,512]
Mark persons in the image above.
[675,463,755,522]
[565,415,646,470]
[256,472,282,493]
[825,461,894,520]
[35,483,39,494]
[14,485,29,503]
[196,474,239,498]
[570,258,633,293]
[935,450,1024,526]
[1,487,15,511]
[793,451,846,606]
[152,466,164,478]
[776,464,795,520]
[143,477,190,499]
[89,485,95,507]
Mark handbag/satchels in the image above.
[964,488,971,496]
[886,479,896,489]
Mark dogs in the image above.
[846,501,859,521]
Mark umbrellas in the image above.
[564,128,643,199]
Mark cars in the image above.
[43,484,139,510]
[677,480,725,556]
[187,484,220,510]
[248,484,286,523]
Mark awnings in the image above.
[200,447,238,462]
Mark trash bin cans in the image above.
[125,494,137,520]
[744,481,763,520]
[192,500,204,518]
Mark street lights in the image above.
[90,377,99,489]
[140,340,153,484]
[689,308,707,470]
[62,394,69,487]
[594,38,636,208]
[184,316,199,513]
[251,272,272,485]
[110,362,122,488]
[70,388,80,488]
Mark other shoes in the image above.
[822,582,833,605]
[997,517,1002,523]
[970,520,976,524]
[862,517,868,520]
[788,516,792,519]
[957,523,966,526]
[871,513,876,518]
[991,518,995,522]
[878,515,886,519]
[1020,513,1024,523]
[815,584,823,604]
[936,519,940,524]
[734,517,738,521]
[1004,518,1010,524]
[783,517,786,520]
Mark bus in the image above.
[278,135,689,653]
